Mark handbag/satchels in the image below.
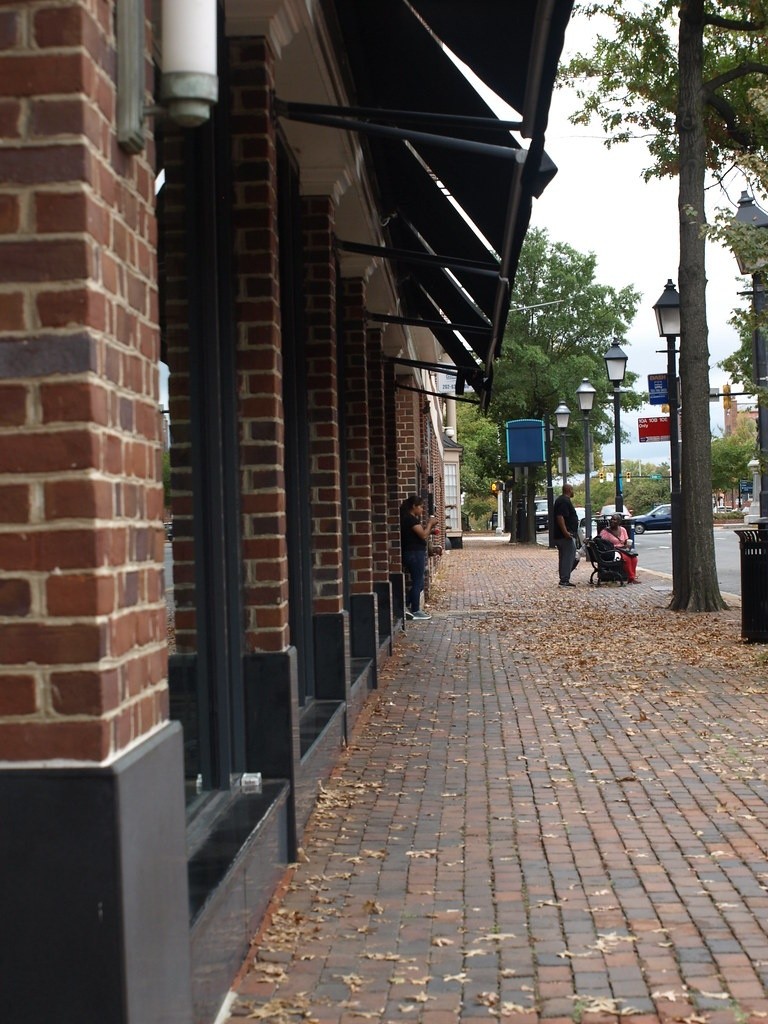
[619,547,638,556]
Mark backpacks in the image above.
[593,536,615,560]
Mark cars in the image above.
[574,507,586,521]
[630,505,672,534]
[596,504,635,527]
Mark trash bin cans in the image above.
[735,530,768,644]
[594,514,636,549]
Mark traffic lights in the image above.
[600,473,603,483]
[626,471,631,481]
[491,483,499,491]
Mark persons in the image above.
[400,496,439,620]
[599,514,641,583]
[553,484,579,589]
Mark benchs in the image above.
[583,537,624,587]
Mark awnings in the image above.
[274,0,572,416]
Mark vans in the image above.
[534,500,549,531]
[718,506,732,512]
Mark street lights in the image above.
[604,338,629,512]
[651,280,681,593]
[576,378,597,547]
[727,189,768,540]
[554,402,571,484]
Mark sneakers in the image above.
[404,607,413,620]
[412,610,431,619]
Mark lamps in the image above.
[118,1,220,148]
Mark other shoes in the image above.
[628,579,641,584]
[558,579,576,588]
